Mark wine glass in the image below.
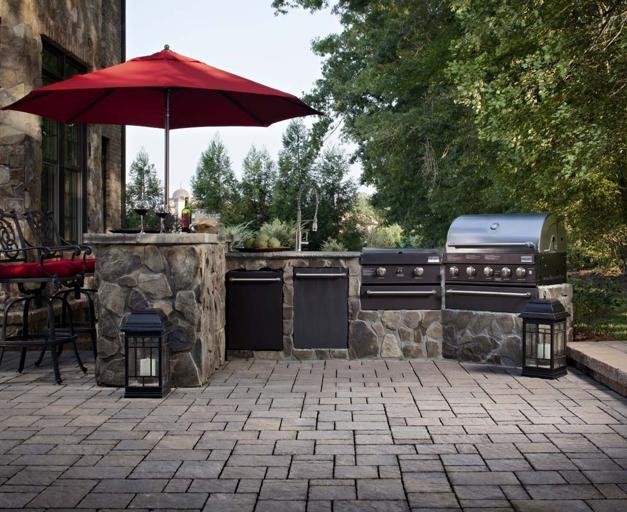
[133,199,150,234]
[154,203,169,234]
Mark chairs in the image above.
[0,209,87,384]
[24,208,98,367]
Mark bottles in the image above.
[182,197,192,232]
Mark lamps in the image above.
[517,290,572,380]
[121,309,170,398]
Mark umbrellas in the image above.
[0,44,325,213]
